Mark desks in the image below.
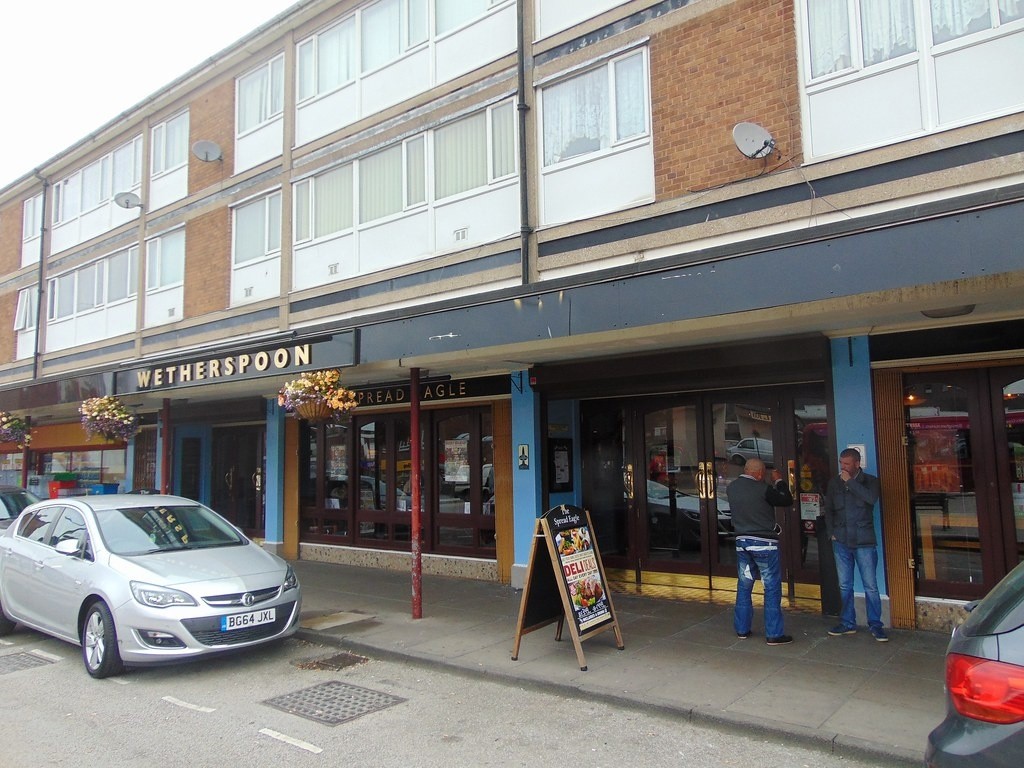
[917,512,1024,582]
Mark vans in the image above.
[726,438,772,468]
[455,464,494,503]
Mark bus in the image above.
[804,411,1024,484]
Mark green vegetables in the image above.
[564,540,572,548]
[576,579,596,606]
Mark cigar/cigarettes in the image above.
[838,471,843,476]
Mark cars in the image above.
[310,475,425,541]
[924,562,1024,768]
[647,479,734,552]
[0,484,304,679]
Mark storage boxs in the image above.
[27,475,119,499]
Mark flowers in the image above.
[78,394,143,445]
[277,369,360,424]
[0,411,37,449]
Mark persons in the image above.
[725,458,794,646]
[824,449,889,641]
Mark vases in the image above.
[295,402,334,424]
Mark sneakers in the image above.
[828,623,856,635]
[766,633,794,645]
[872,627,889,642]
[738,630,752,639]
